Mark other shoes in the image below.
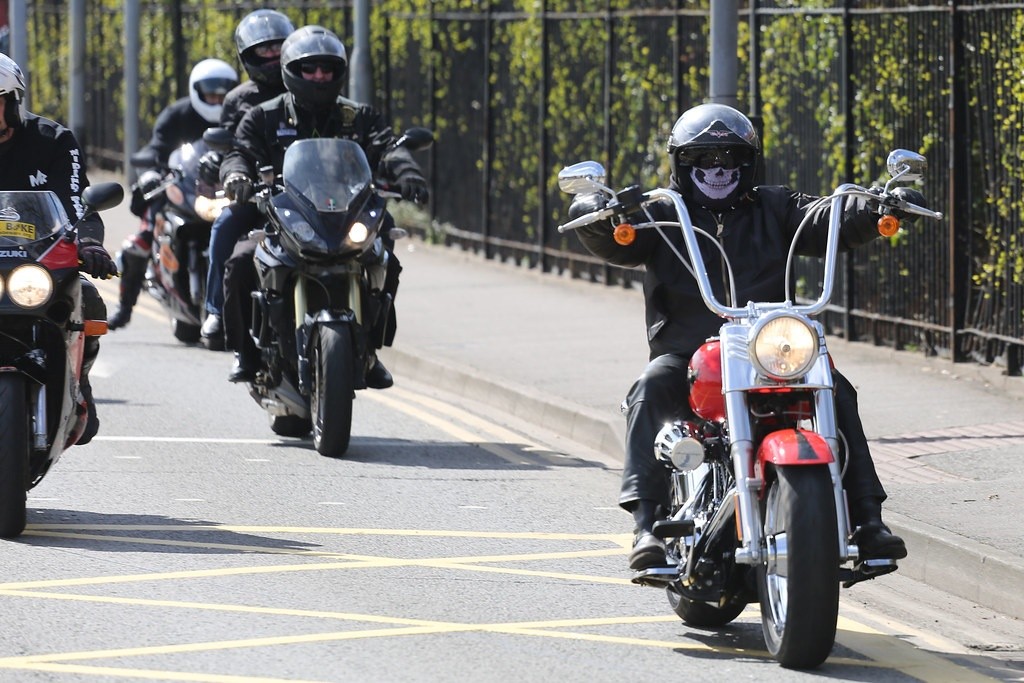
[107,311,130,330]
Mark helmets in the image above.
[0,53,26,105]
[667,103,761,183]
[189,58,237,123]
[280,25,348,103]
[234,9,294,86]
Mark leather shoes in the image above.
[228,367,247,382]
[200,313,222,338]
[628,520,667,569]
[365,357,393,389]
[849,519,907,559]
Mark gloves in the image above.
[198,151,223,186]
[78,245,118,280]
[890,187,926,223]
[222,171,252,204]
[569,189,615,238]
[142,179,162,199]
[393,169,429,204]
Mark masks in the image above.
[689,166,744,199]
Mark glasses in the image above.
[297,60,334,74]
[254,44,281,55]
[678,148,749,170]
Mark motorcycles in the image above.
[130,139,226,353]
[558,149,944,670]
[202,127,436,458]
[0,181,125,538]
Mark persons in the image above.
[219,28,430,389]
[200,9,294,339]
[0,53,118,446]
[105,58,239,331]
[569,104,926,569]
